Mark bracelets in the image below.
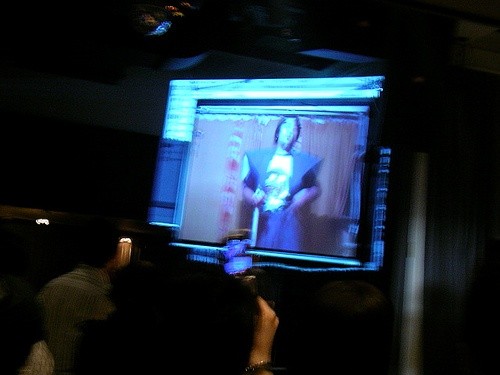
[244,358,272,374]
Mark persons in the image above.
[108,255,281,375]
[238,114,323,254]
[37,222,123,375]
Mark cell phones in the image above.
[222,228,254,288]
